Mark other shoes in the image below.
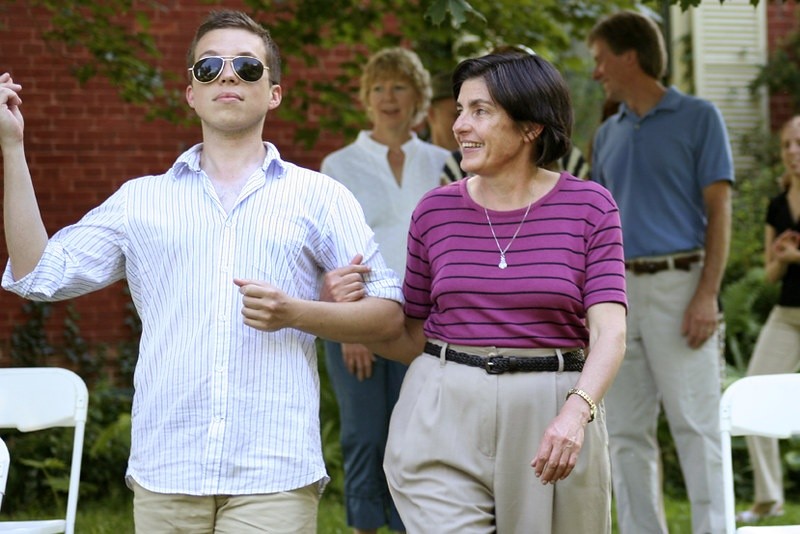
[733,501,787,523]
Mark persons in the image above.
[437,10,734,534]
[0,9,406,534]
[321,47,449,534]
[320,43,628,534]
[738,116,800,524]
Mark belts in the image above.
[625,254,702,273]
[423,341,586,375]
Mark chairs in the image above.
[0,366,89,534]
[720,373,800,534]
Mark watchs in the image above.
[564,386,598,424]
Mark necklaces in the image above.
[475,173,533,270]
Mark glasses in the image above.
[187,55,271,84]
